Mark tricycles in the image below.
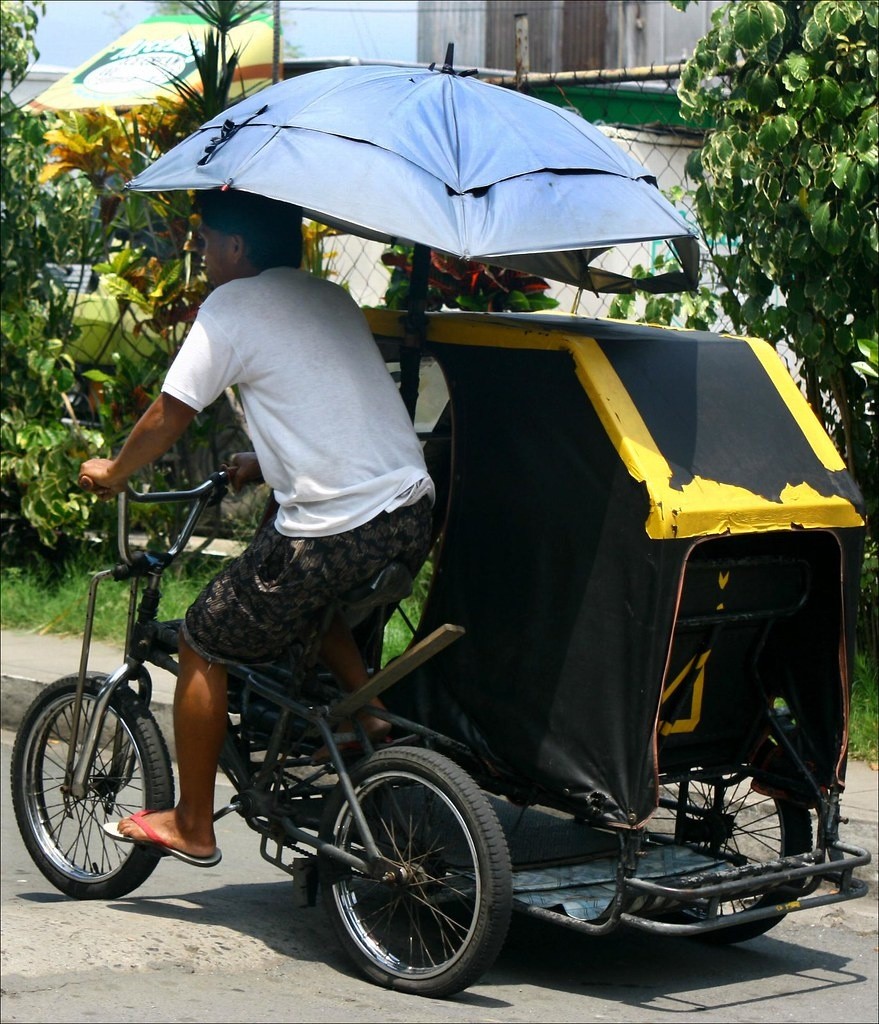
[9,38,871,998]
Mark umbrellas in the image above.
[122,42,703,434]
[18,11,281,205]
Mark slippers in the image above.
[102,809,222,867]
[310,733,418,765]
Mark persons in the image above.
[73,189,437,867]
